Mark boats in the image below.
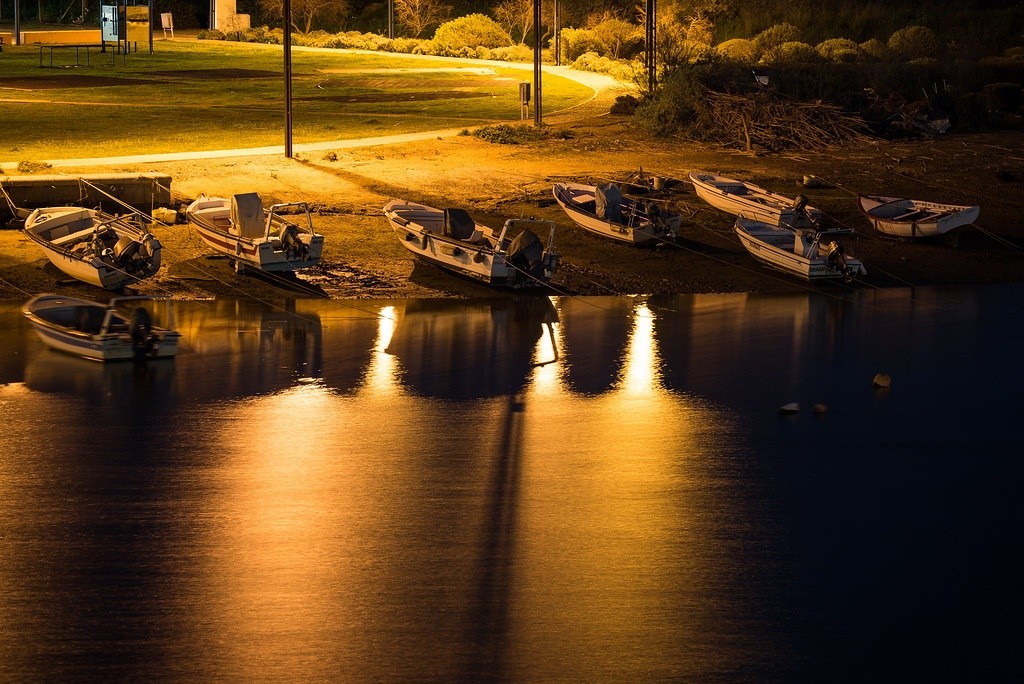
[23,205,162,292]
[186,192,325,272]
[688,170,823,231]
[856,193,979,249]
[553,181,683,245]
[25,347,172,405]
[733,209,855,284]
[21,291,180,364]
[383,197,562,289]
